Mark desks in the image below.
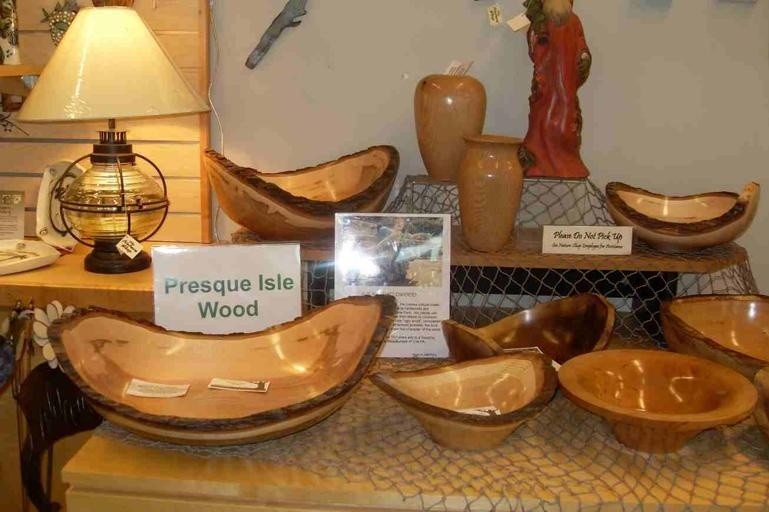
[231,224,747,312]
[60,307,769,512]
[0,239,229,512]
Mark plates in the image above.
[0,239,62,277]
[203,146,401,240]
[371,352,558,452]
[47,294,397,446]
[35,159,87,253]
[605,182,761,252]
[660,293,769,384]
[441,294,616,374]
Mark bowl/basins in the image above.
[558,350,758,455]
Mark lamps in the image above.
[16,6,212,274]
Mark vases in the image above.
[414,74,487,184]
[457,135,524,253]
